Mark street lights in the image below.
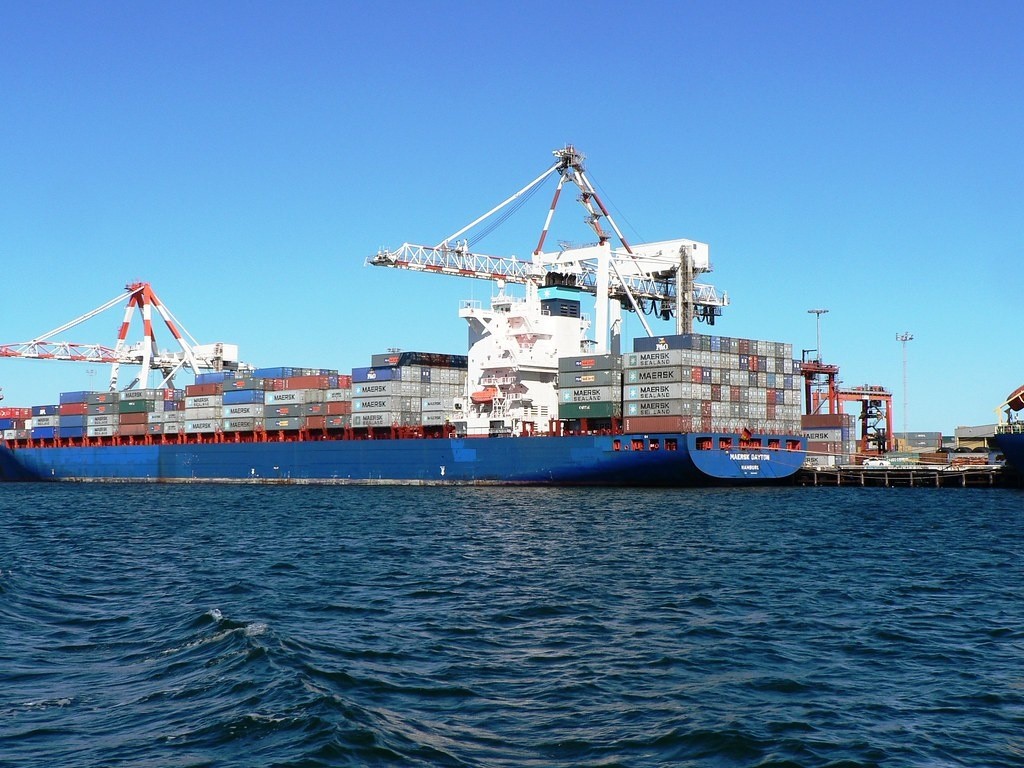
[896,332,915,451]
[808,308,829,363]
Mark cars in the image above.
[862,457,890,466]
[936,447,954,453]
[973,447,990,453]
[954,447,973,453]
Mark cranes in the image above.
[365,141,731,355]
[0,278,257,394]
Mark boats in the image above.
[994,385,1024,482]
[0,430,808,486]
